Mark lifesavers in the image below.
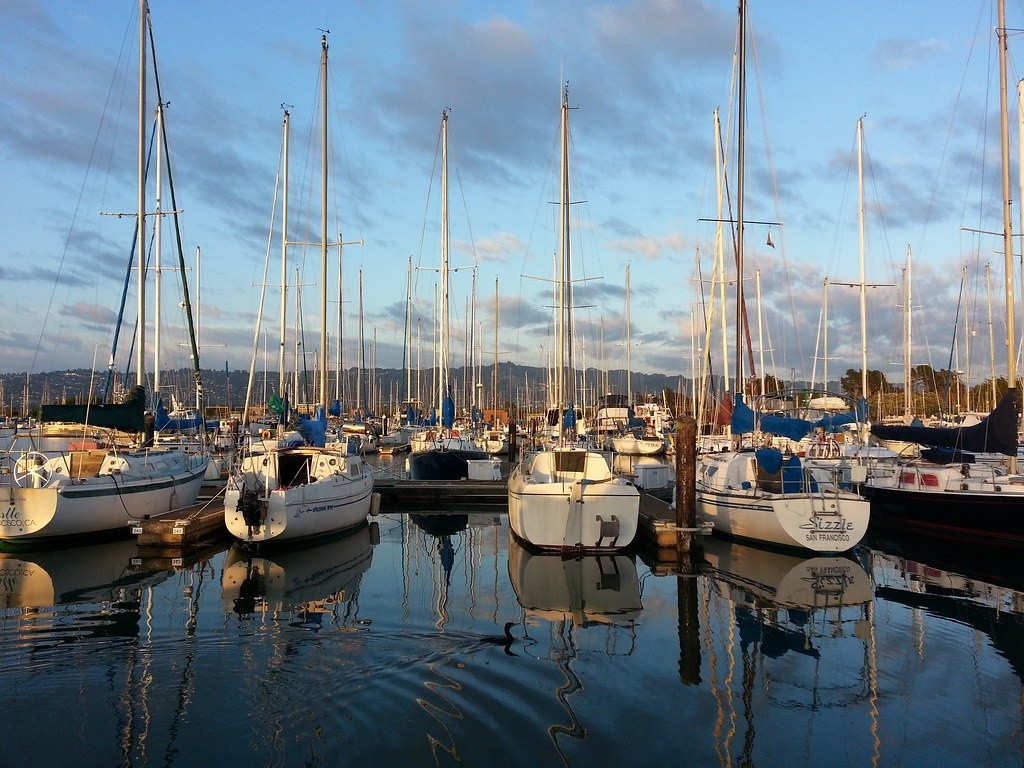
[262,430,271,439]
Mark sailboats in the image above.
[698,535,875,738]
[508,81,640,554]
[0,0,208,536]
[223,523,378,765]
[508,540,643,765]
[3,538,173,766]
[672,0,870,552]
[225,34,377,541]
[0,5,1018,498]
[837,117,1024,532]
